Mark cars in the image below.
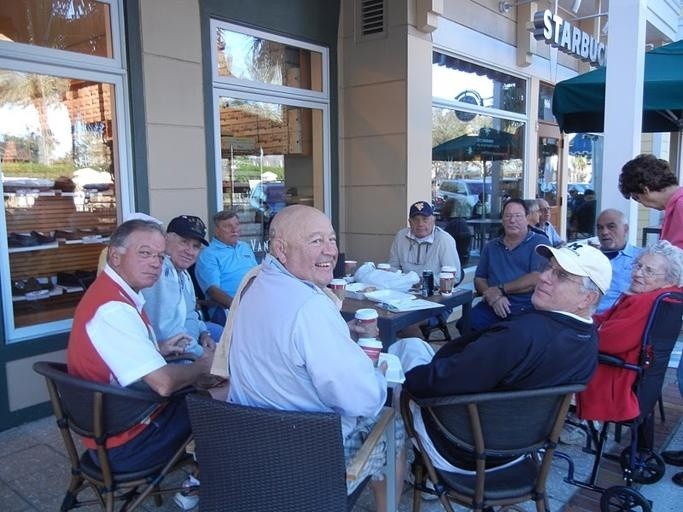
[431,178,592,217]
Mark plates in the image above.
[340,283,385,301]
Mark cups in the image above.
[437,264,456,297]
[343,261,359,278]
[329,278,347,293]
[354,307,384,368]
[377,263,392,272]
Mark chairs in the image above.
[451,232,474,265]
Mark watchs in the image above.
[497,284,507,297]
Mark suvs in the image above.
[2,177,78,303]
[250,180,300,220]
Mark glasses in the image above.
[629,261,668,278]
[536,260,581,285]
[500,212,526,222]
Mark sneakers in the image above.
[174,474,202,509]
[403,459,441,502]
[559,415,601,443]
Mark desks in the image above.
[642,225,662,248]
[465,218,503,256]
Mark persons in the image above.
[565,239,682,425]
[389,200,463,342]
[96,212,163,281]
[455,198,553,337]
[660,355,682,486]
[522,200,550,243]
[617,153,683,250]
[194,211,259,328]
[65,217,217,510]
[388,243,614,502]
[135,215,216,371]
[533,199,561,248]
[226,203,415,512]
[552,208,647,446]
[471,186,597,243]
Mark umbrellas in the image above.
[551,37,683,188]
[432,128,559,197]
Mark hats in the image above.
[408,199,434,219]
[165,214,211,247]
[533,240,613,294]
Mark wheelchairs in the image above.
[545,288,683,512]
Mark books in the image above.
[376,298,445,313]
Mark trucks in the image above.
[70,168,115,212]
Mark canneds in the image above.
[421,270,435,298]
